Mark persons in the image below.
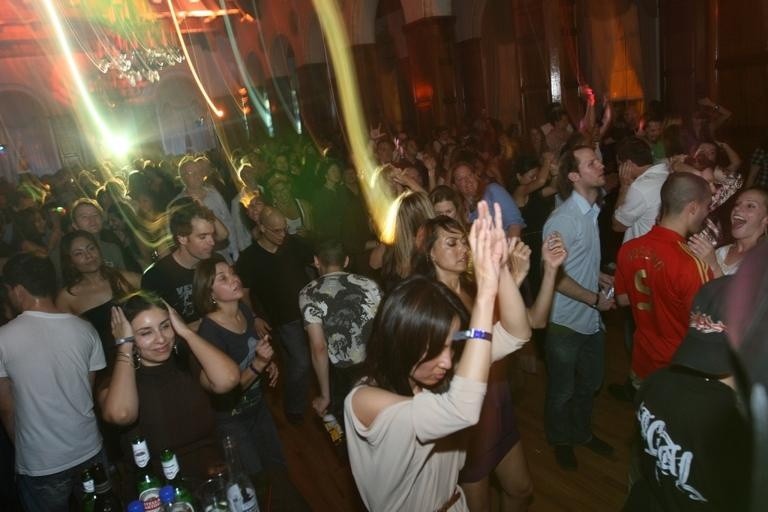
[1,83,768,511]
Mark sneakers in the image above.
[608,385,636,401]
[583,434,614,456]
[555,437,577,471]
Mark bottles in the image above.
[77,430,261,512]
[319,409,346,447]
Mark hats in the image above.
[670,274,736,377]
